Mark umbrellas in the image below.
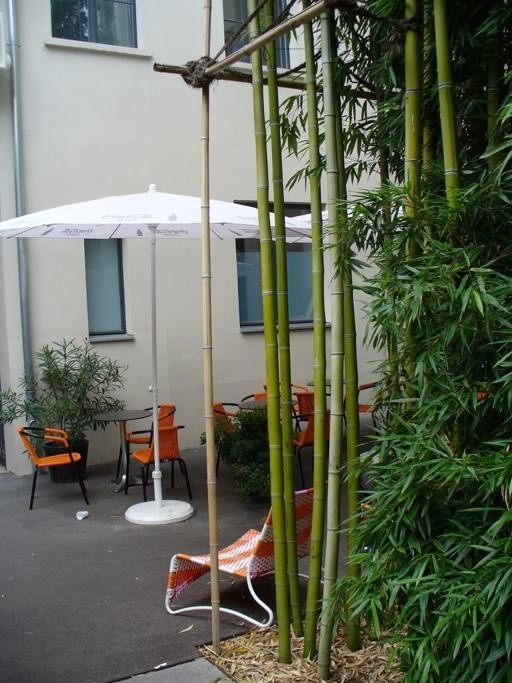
[0,183,317,501]
[289,203,407,223]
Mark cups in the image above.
[75,510,89,521]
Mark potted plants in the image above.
[4,336,128,483]
[200,404,281,504]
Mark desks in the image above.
[307,378,346,432]
[95,409,153,492]
[239,399,299,410]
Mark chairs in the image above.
[166,480,327,628]
[263,383,313,432]
[292,409,330,489]
[343,382,391,438]
[125,426,192,502]
[241,393,281,403]
[213,403,251,479]
[16,426,89,510]
[116,405,185,486]
